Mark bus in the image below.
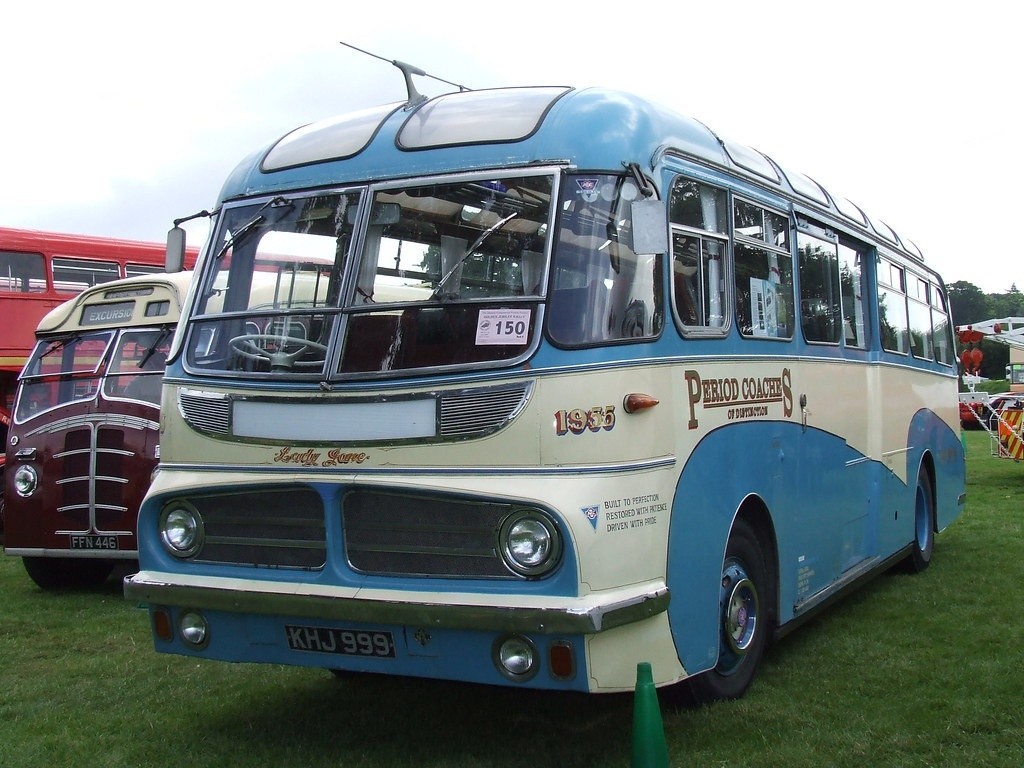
[0,269,437,593]
[122,83,968,704]
[1004,346,1024,393]
[0,226,337,474]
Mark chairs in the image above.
[341,315,423,374]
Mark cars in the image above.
[959,392,1024,431]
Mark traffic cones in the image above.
[628,662,672,768]
